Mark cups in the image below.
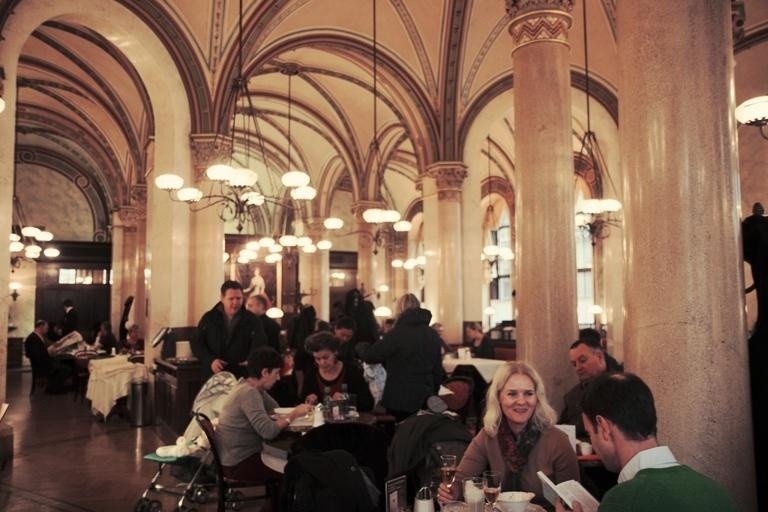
[463,476,487,510]
[443,500,468,511]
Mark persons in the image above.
[189,281,448,422]
[555,370,742,512]
[55,300,88,347]
[122,331,145,354]
[241,268,266,297]
[23,319,76,392]
[435,360,581,511]
[95,322,120,355]
[465,322,495,359]
[556,336,618,437]
[213,347,302,512]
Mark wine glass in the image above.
[439,453,456,488]
[483,470,504,511]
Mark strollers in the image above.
[134,371,247,510]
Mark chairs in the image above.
[19,341,626,511]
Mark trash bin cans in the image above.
[130,382,154,426]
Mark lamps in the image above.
[573,1,622,248]
[479,131,516,268]
[154,1,320,233]
[224,233,333,270]
[737,95,768,143]
[323,1,413,255]
[391,256,430,271]
[9,82,59,301]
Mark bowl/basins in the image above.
[497,491,535,510]
[578,443,593,455]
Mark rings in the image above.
[437,494,440,498]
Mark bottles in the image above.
[323,386,350,421]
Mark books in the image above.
[536,469,600,512]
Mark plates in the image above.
[273,407,298,415]
